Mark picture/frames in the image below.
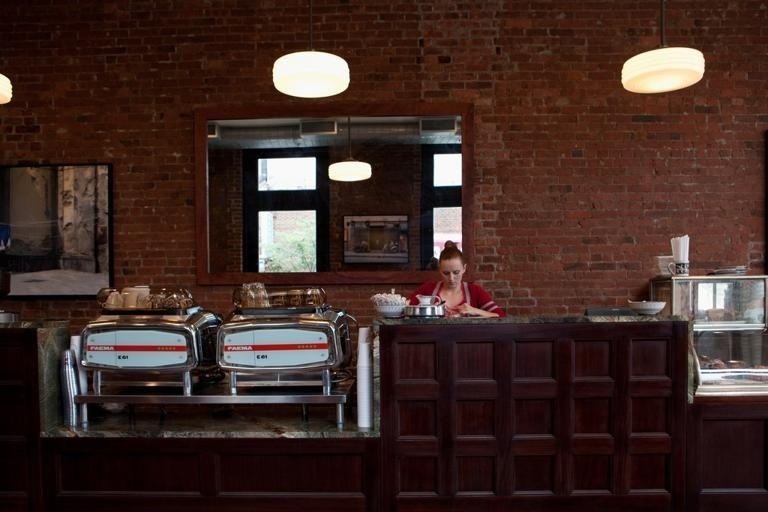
[339,212,411,267]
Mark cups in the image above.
[96,284,151,310]
[355,326,373,432]
[416,294,441,306]
[667,259,690,276]
[231,281,271,309]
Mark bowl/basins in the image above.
[375,305,407,318]
[627,297,667,316]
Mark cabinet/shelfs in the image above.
[648,275,767,512]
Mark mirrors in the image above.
[3,162,114,302]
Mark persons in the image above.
[403,240,507,320]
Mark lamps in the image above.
[327,116,373,183]
[620,0,705,95]
[271,0,352,99]
